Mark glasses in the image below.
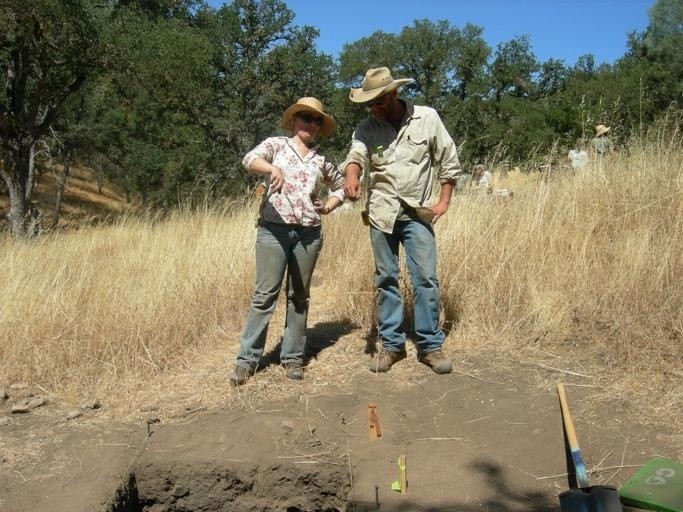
[294,112,323,126]
[365,94,391,108]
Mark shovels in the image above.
[556,383,622,512]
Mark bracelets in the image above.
[323,203,330,214]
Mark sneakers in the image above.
[417,348,453,375]
[284,362,304,380]
[369,347,407,373]
[228,366,251,385]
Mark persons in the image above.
[230,97,348,386]
[337,66,462,374]
[469,163,493,190]
[567,136,590,173]
[587,125,615,169]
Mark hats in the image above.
[281,96,336,137]
[594,125,610,138]
[347,67,410,104]
